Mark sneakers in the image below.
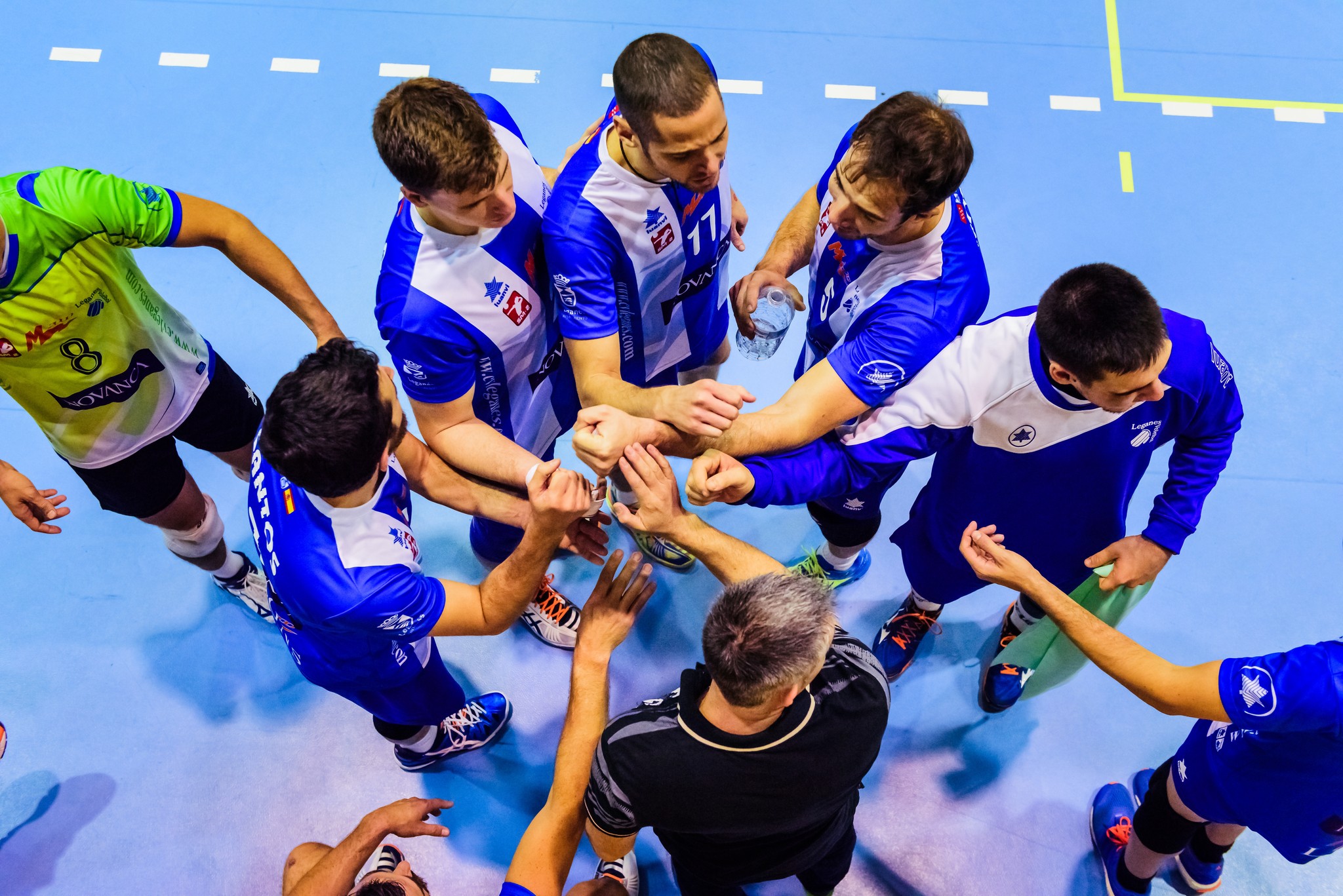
[210,550,275,623]
[788,543,871,591]
[354,844,405,886]
[594,851,639,896]
[873,586,943,680]
[393,690,513,770]
[606,485,697,570]
[1128,768,1224,893]
[1089,780,1151,896]
[518,574,581,648]
[981,599,1035,708]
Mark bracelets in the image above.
[525,464,539,485]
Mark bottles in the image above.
[739,286,796,362]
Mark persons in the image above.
[0,166,347,623]
[960,520,1343,896]
[245,33,990,894]
[684,261,1244,711]
